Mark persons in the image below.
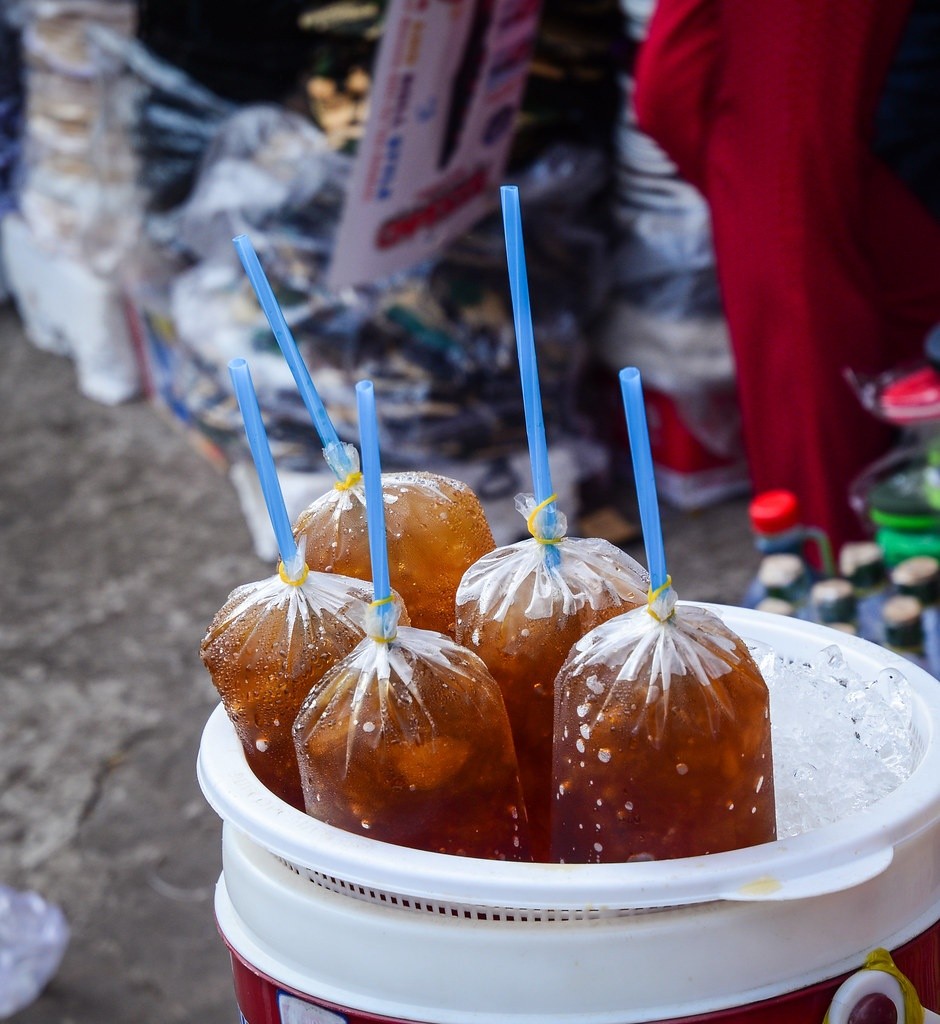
[632,0,940,576]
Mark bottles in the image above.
[736,487,940,683]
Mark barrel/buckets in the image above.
[196,604,938,1024]
